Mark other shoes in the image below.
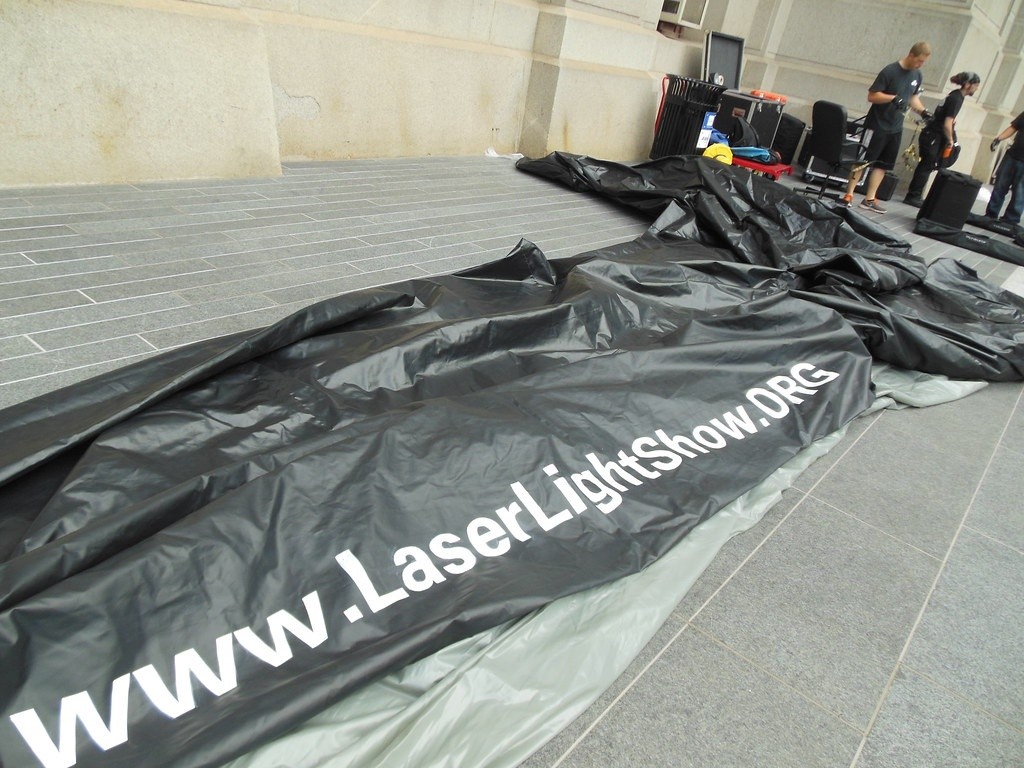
[903,196,925,208]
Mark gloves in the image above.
[891,94,907,111]
[990,138,1000,151]
[919,108,934,123]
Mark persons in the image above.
[842,41,936,214]
[902,71,981,208]
[984,111,1024,225]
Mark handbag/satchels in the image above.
[903,118,922,172]
[934,122,960,169]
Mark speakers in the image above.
[917,170,982,230]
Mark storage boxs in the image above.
[699,29,787,150]
[796,126,870,186]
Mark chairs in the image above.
[794,101,870,208]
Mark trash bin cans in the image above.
[648,74,728,161]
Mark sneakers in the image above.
[843,193,853,207]
[858,198,887,213]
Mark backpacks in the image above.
[730,145,778,165]
[727,115,761,148]
